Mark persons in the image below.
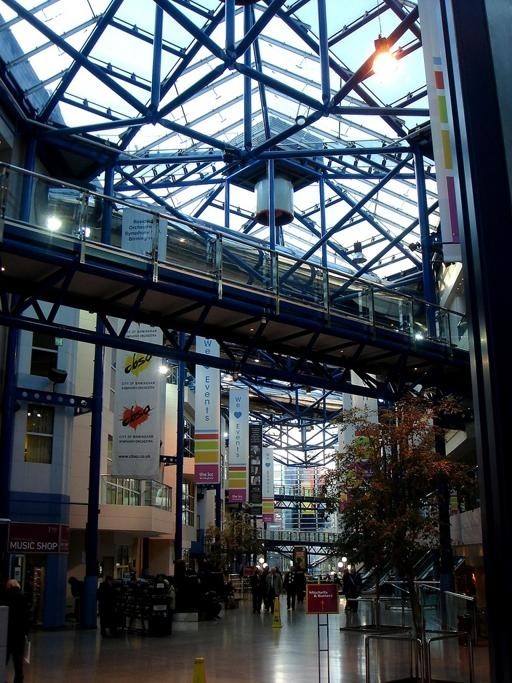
[381,579,395,610]
[1,579,37,683]
[68,562,364,639]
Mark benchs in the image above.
[96,611,153,640]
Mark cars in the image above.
[115,576,150,616]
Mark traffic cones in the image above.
[191,657,209,682]
[272,596,283,627]
